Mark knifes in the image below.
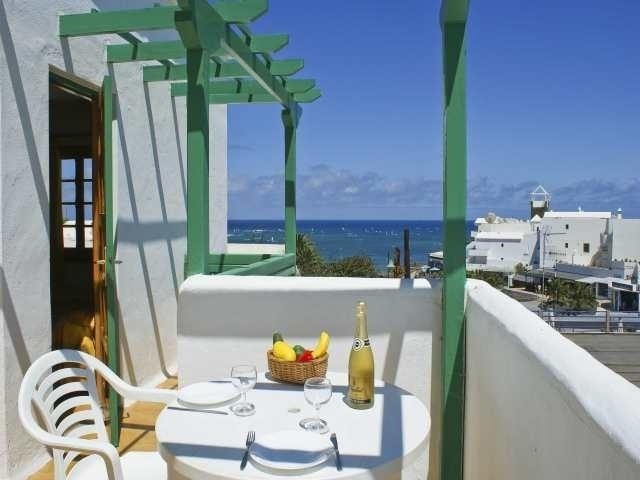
[330,434,343,472]
[167,405,230,416]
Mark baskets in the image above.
[267,349,329,385]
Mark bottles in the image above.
[346,305,375,411]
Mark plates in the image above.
[177,383,240,406]
[249,429,334,471]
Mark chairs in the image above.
[17,348,178,480]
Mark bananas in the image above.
[311,329,330,358]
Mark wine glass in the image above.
[301,378,332,429]
[230,365,257,417]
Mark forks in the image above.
[240,430,254,469]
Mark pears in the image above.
[272,340,297,361]
